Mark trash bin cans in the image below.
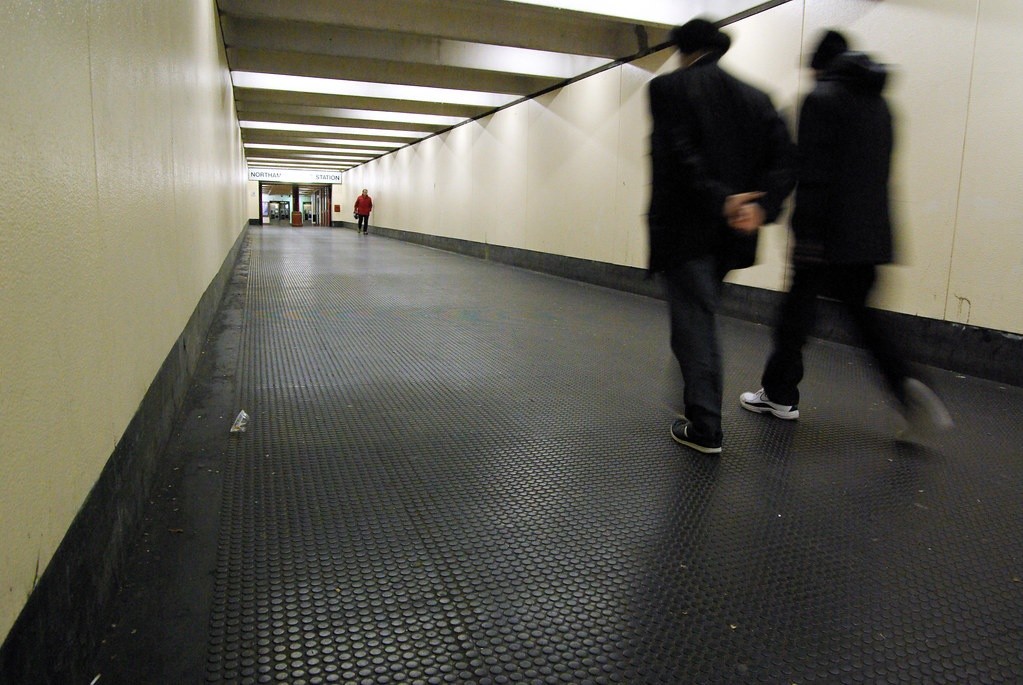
[292,211,303,227]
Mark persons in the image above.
[740,30,952,441]
[353,189,372,235]
[647,20,798,453]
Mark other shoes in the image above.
[363,230,368,235]
[357,226,361,233]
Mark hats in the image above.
[812,31,847,68]
[669,19,732,53]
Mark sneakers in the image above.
[670,418,722,453]
[896,413,953,444]
[740,387,799,419]
